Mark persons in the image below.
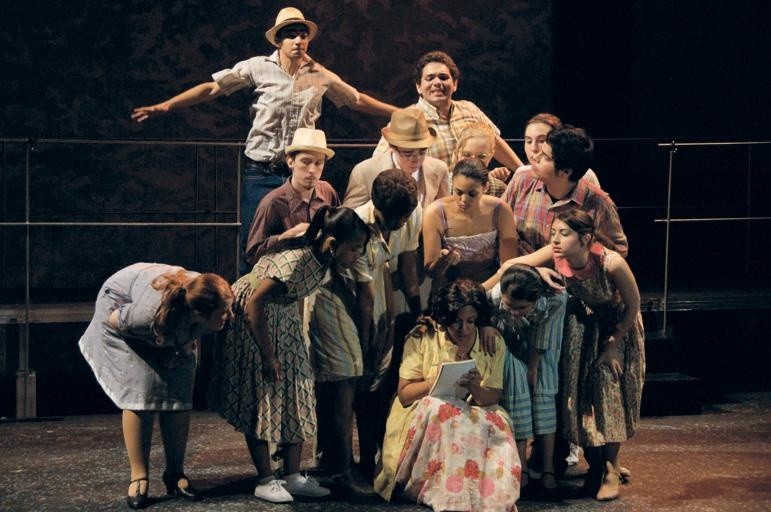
[480,115,645,500]
[374,273,522,511]
[77,262,234,510]
[370,50,524,171]
[210,127,425,503]
[348,113,518,302]
[130,6,401,275]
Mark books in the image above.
[430,359,477,399]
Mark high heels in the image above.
[162,470,204,501]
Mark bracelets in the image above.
[172,97,178,107]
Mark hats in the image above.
[382,107,439,150]
[283,127,336,162]
[265,7,319,48]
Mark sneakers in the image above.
[283,471,330,497]
[126,471,150,508]
[255,479,293,503]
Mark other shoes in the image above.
[520,462,631,501]
[328,471,378,501]
